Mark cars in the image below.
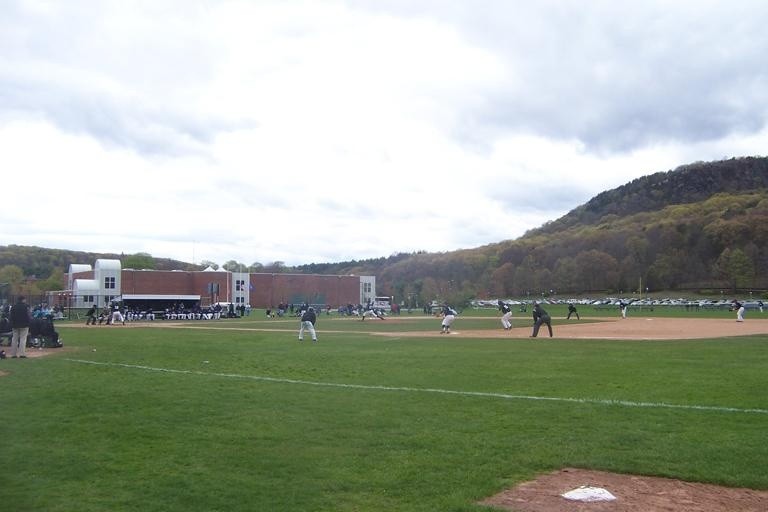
[471,297,767,308]
[427,300,448,307]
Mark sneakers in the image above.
[439,329,450,334]
[504,326,513,330]
[11,354,27,360]
[529,334,536,338]
[298,338,305,341]
[313,338,318,341]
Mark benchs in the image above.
[0,331,49,347]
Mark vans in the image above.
[209,301,235,309]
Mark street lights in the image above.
[68,292,71,320]
[62,293,65,307]
[58,293,61,305]
[719,290,724,298]
[541,292,544,296]
[749,292,752,298]
[550,289,553,296]
[527,292,530,299]
[646,287,649,302]
[391,295,394,304]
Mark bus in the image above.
[375,296,391,307]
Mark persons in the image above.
[497,301,513,330]
[759,301,763,312]
[731,299,744,321]
[439,302,456,334]
[566,303,579,320]
[620,303,627,318]
[530,304,553,337]
